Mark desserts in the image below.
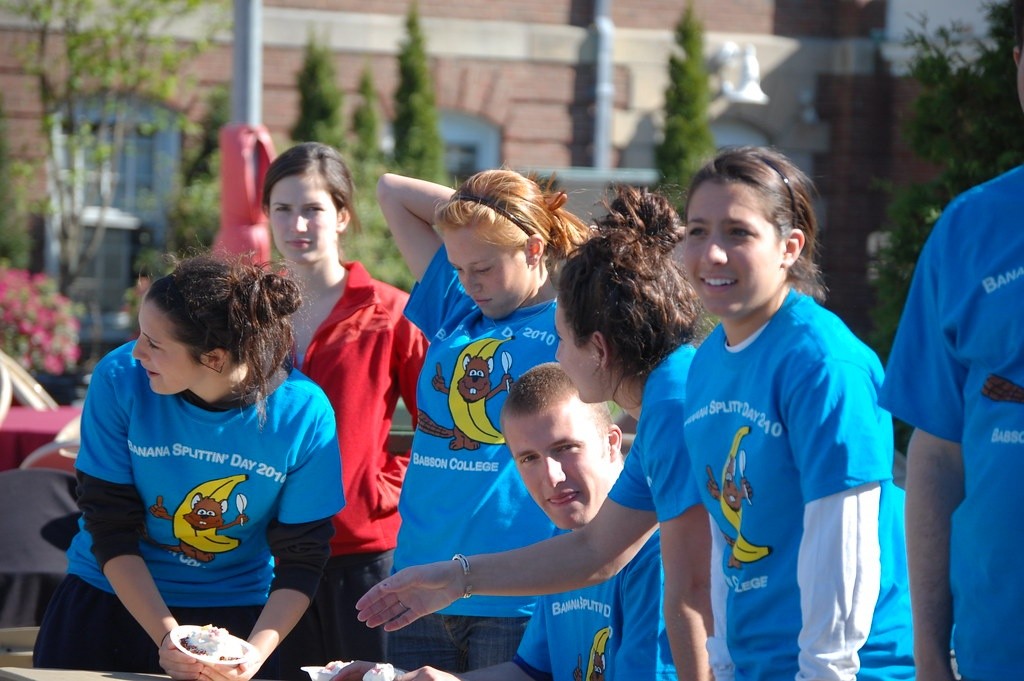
[317,660,350,674]
[361,662,399,681]
[180,623,243,661]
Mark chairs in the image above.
[0,464,81,627]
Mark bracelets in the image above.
[452,553,473,600]
[161,630,171,647]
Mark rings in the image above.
[398,601,407,612]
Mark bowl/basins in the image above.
[300,666,334,681]
[170,624,256,670]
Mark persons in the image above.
[245,142,723,681]
[682,145,914,679]
[878,1,1023,681]
[33,247,345,679]
